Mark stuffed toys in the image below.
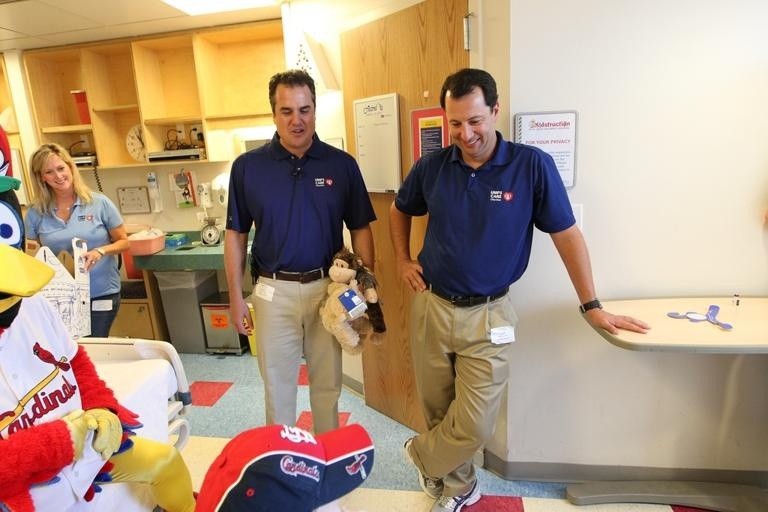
[0,176,198,512]
[319,279,373,355]
[328,248,386,334]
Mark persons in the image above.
[222,70,378,434]
[23,143,132,340]
[389,68,651,512]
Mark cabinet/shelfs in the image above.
[18,16,289,172]
[102,224,169,342]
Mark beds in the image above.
[49,335,193,511]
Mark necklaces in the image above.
[66,199,74,210]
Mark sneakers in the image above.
[403,436,444,499]
[429,475,481,512]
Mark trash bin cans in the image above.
[153,269,218,353]
[199,291,249,356]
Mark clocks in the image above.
[124,122,148,163]
[197,217,225,248]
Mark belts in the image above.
[421,277,510,307]
[259,268,329,284]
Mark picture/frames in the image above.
[115,184,152,216]
[352,92,403,194]
[513,108,579,191]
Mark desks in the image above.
[132,230,257,273]
[563,287,767,512]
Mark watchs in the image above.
[92,247,106,257]
[579,298,603,313]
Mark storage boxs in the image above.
[240,292,259,356]
[124,233,167,257]
